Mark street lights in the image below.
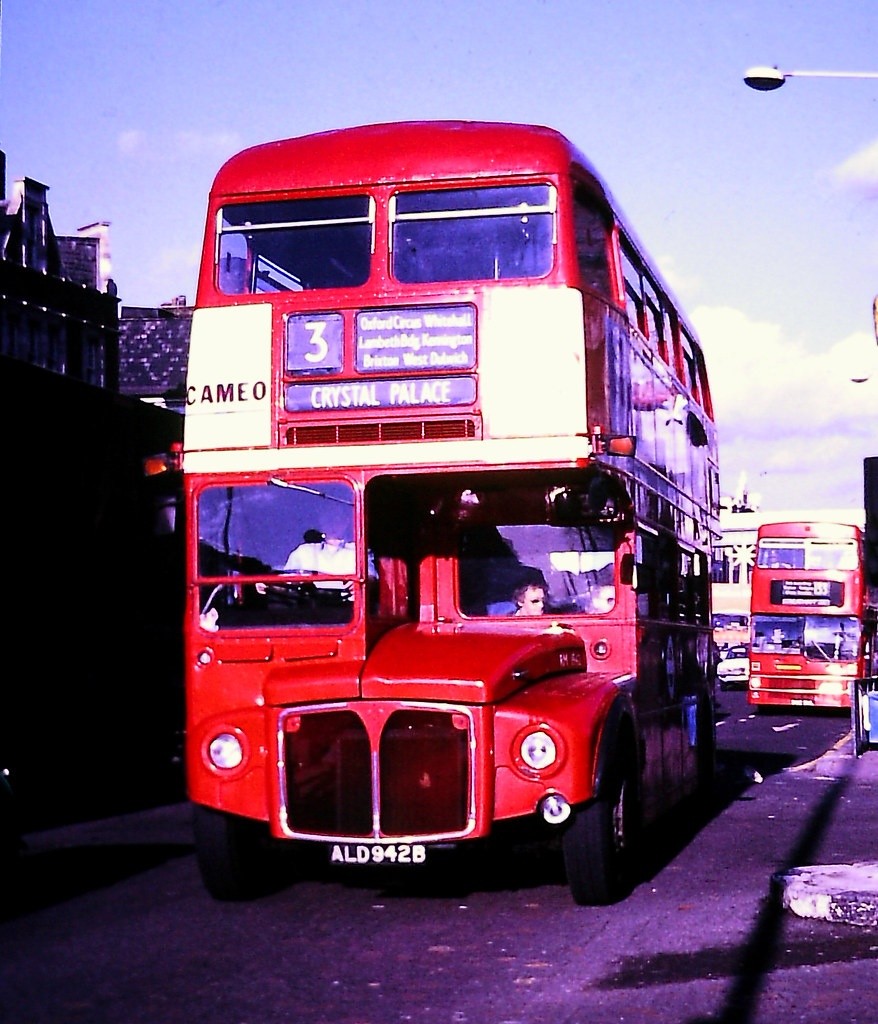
[738,64,877,95]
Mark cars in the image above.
[716,646,751,690]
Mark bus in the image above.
[747,521,866,712]
[140,121,725,910]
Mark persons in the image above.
[496,196,550,279]
[583,584,618,615]
[506,576,552,616]
